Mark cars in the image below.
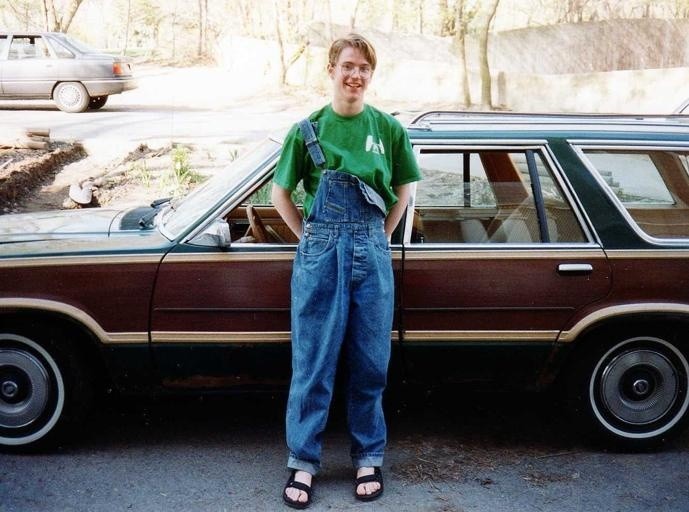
[0,31,139,111]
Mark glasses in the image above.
[341,63,374,80]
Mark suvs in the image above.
[0,104,689,448]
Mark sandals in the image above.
[283,469,313,508]
[356,466,384,501]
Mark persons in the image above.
[271,33,410,508]
